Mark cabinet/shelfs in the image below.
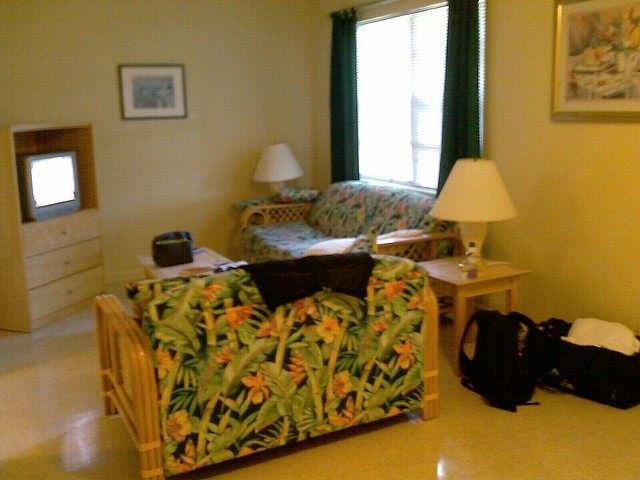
[1,124,108,333]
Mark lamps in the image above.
[252,142,303,201]
[429,157,519,267]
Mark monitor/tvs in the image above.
[20,149,87,222]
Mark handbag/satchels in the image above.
[535,316,640,411]
[151,231,193,267]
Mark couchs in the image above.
[94,252,440,476]
[241,178,465,325]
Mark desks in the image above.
[138,247,235,286]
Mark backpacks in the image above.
[457,307,541,412]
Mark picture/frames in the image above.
[550,0,640,125]
[116,62,187,120]
